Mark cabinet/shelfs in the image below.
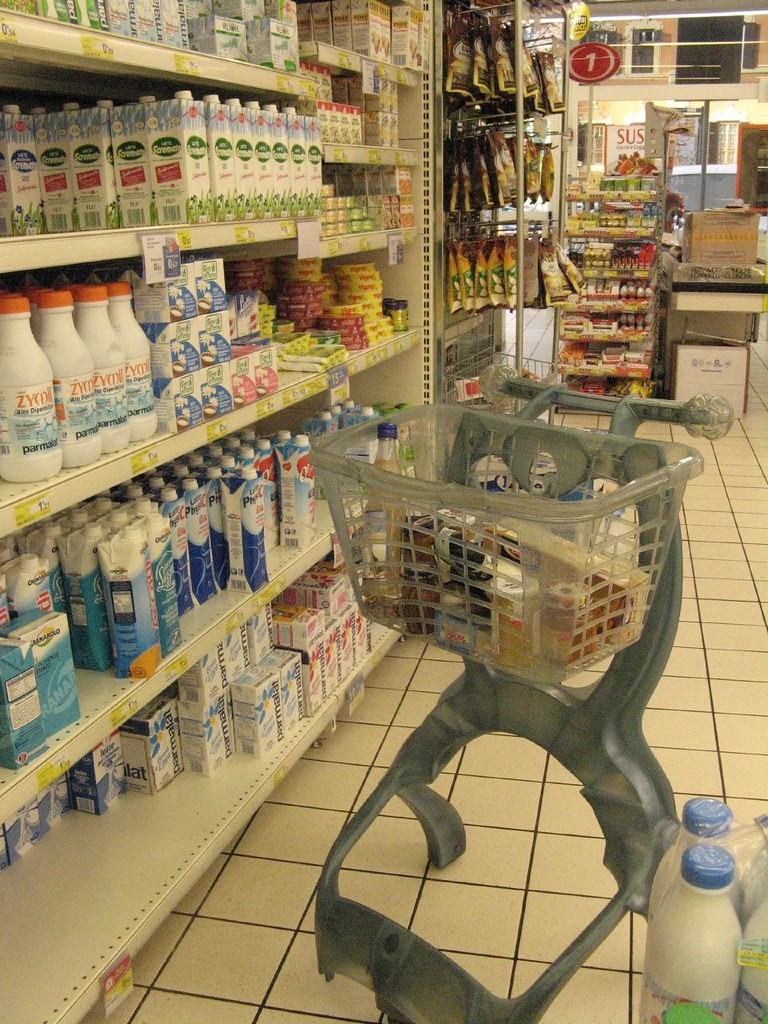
[0,2,434,1024]
[442,1,670,406]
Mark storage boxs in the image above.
[682,210,760,266]
[0,0,430,237]
[672,330,750,421]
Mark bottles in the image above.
[360,423,406,599]
[543,583,594,665]
[0,399,413,678]
[637,795,768,1024]
[489,575,542,669]
[0,89,323,226]
[434,594,477,658]
[0,282,157,483]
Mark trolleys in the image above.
[307,360,733,1022]
